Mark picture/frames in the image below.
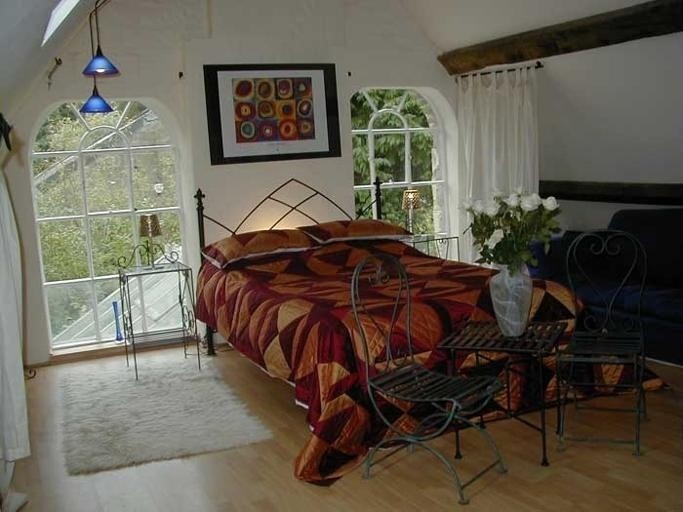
[201,61,342,166]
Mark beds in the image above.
[192,177,665,485]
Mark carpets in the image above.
[56,353,271,478]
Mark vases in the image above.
[485,258,532,339]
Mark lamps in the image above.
[76,20,112,113]
[400,186,427,235]
[82,0,115,80]
[138,214,161,268]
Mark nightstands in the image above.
[115,243,202,380]
[408,233,461,263]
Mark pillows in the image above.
[198,228,315,271]
[300,216,404,243]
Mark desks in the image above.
[443,322,565,465]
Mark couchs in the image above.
[528,209,681,367]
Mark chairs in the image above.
[342,246,505,503]
[559,222,651,455]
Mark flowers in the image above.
[455,177,565,275]
